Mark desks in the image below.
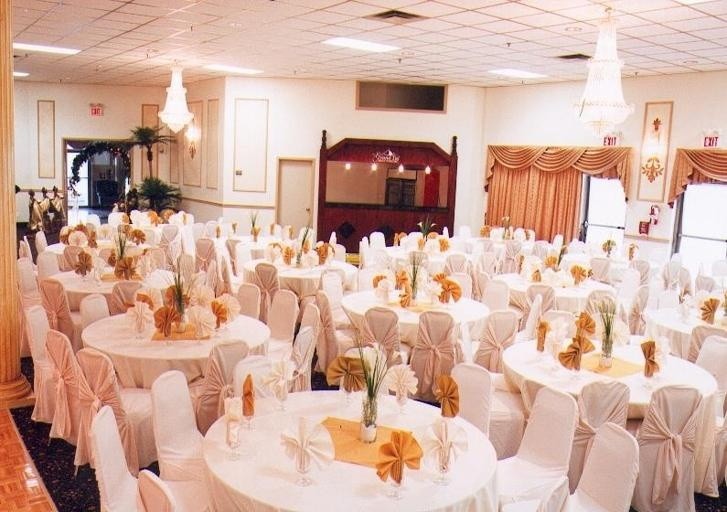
[203,390,497,511]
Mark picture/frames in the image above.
[635,100,675,206]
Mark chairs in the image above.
[232,353,290,398]
[449,361,491,441]
[497,420,641,512]
[695,335,727,414]
[69,348,160,478]
[497,387,581,502]
[703,409,727,498]
[87,404,141,512]
[491,390,523,462]
[569,381,630,497]
[147,370,210,481]
[138,466,184,512]
[14,209,727,384]
[341,346,390,396]
[632,385,706,512]
[40,326,81,448]
[13,182,727,512]
[196,340,248,436]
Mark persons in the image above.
[16,184,32,247]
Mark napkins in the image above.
[377,426,422,485]
[423,420,469,473]
[383,363,418,404]
[327,357,367,394]
[277,415,335,471]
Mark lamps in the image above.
[574,4,637,137]
[156,50,198,137]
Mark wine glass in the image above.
[221,382,456,501]
[132,319,232,347]
